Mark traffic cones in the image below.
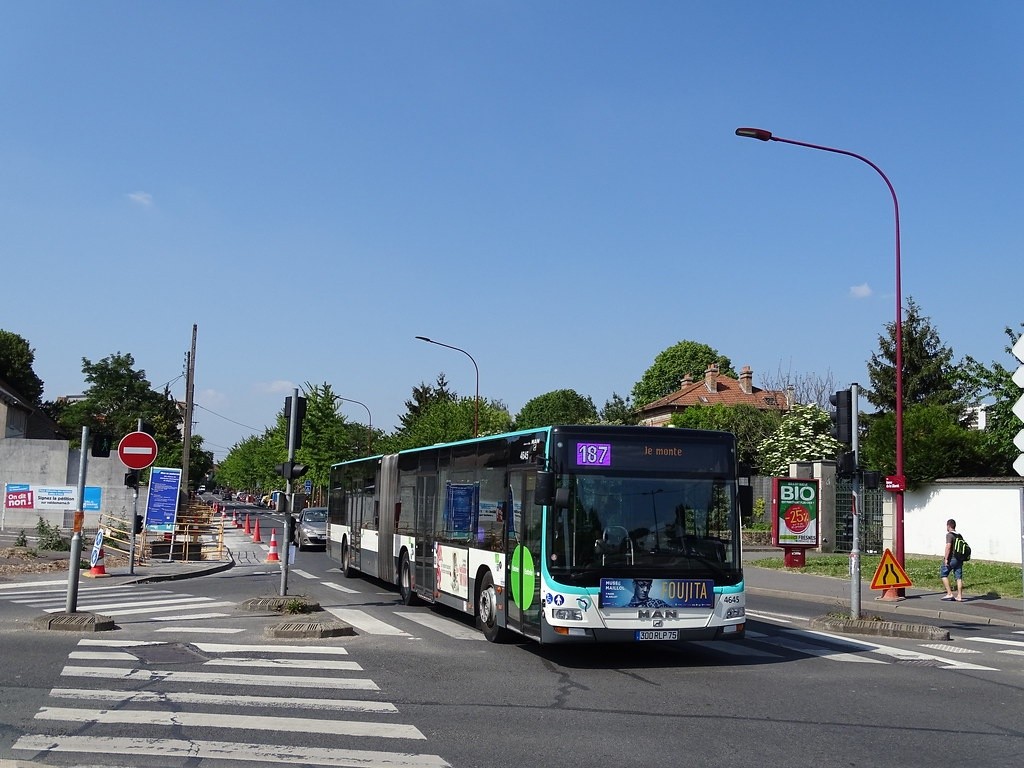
[250,518,265,545]
[242,514,253,536]
[263,528,281,563]
[231,509,238,526]
[221,505,227,519]
[236,512,245,530]
[86,547,112,578]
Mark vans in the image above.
[198,484,206,493]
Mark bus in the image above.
[326,425,755,645]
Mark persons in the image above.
[643,512,684,550]
[940,519,963,601]
[627,580,670,608]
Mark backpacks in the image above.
[950,533,971,561]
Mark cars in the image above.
[219,488,273,510]
[211,489,219,495]
[294,507,327,552]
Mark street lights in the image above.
[415,335,478,438]
[336,395,372,456]
[734,126,905,602]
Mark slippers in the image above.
[941,596,955,601]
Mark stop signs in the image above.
[117,431,158,470]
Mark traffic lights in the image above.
[830,390,853,444]
[134,515,144,534]
[289,462,310,479]
[124,466,140,488]
[91,433,113,458]
[274,462,287,478]
